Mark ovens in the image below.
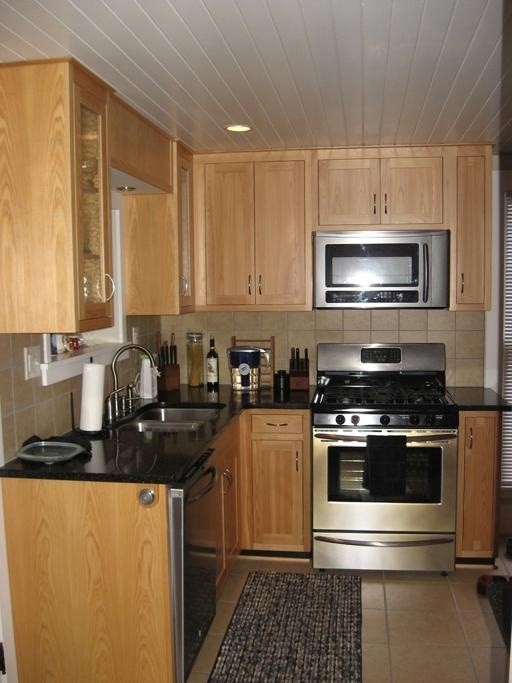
[311,429,457,576]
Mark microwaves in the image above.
[312,229,449,310]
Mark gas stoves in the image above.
[310,387,459,428]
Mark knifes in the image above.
[289,348,308,368]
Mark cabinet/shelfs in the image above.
[456,411,498,570]
[241,409,310,552]
[105,87,169,195]
[214,409,242,561]
[193,149,312,316]
[1,57,115,335]
[4,407,214,678]
[451,146,493,310]
[173,139,195,313]
[313,143,446,229]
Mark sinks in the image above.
[134,405,215,423]
[118,422,202,433]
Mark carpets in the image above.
[209,569,364,681]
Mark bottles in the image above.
[274,370,290,393]
[206,335,219,390]
[186,332,204,387]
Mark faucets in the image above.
[110,343,161,416]
